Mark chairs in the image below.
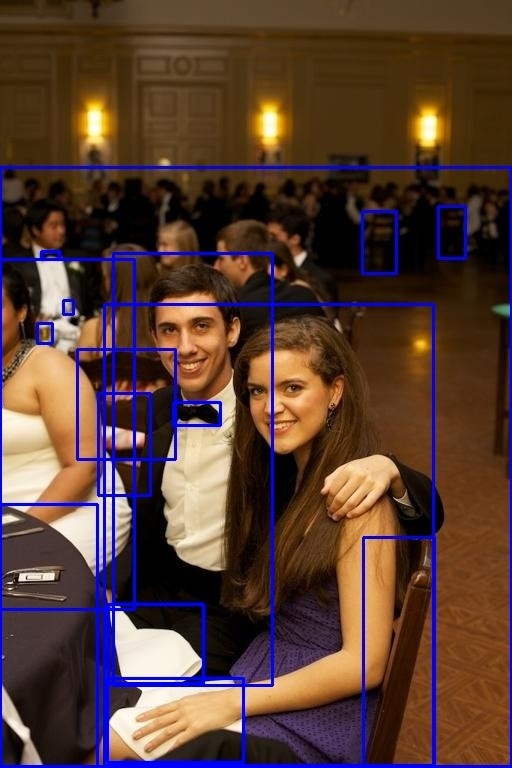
[77,350,176,459]
[439,206,465,259]
[98,394,151,496]
[363,538,435,768]
[362,212,396,273]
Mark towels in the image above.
[109,604,203,683]
[107,678,244,763]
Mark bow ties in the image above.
[174,402,220,425]
[41,252,59,259]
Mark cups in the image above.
[37,323,53,344]
[63,301,73,315]
